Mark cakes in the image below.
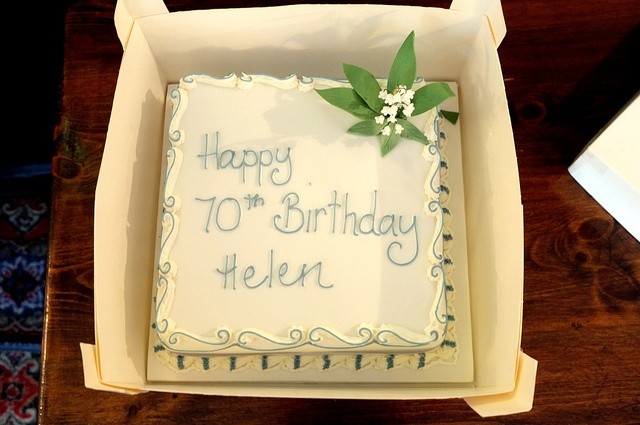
[151,30,458,374]
